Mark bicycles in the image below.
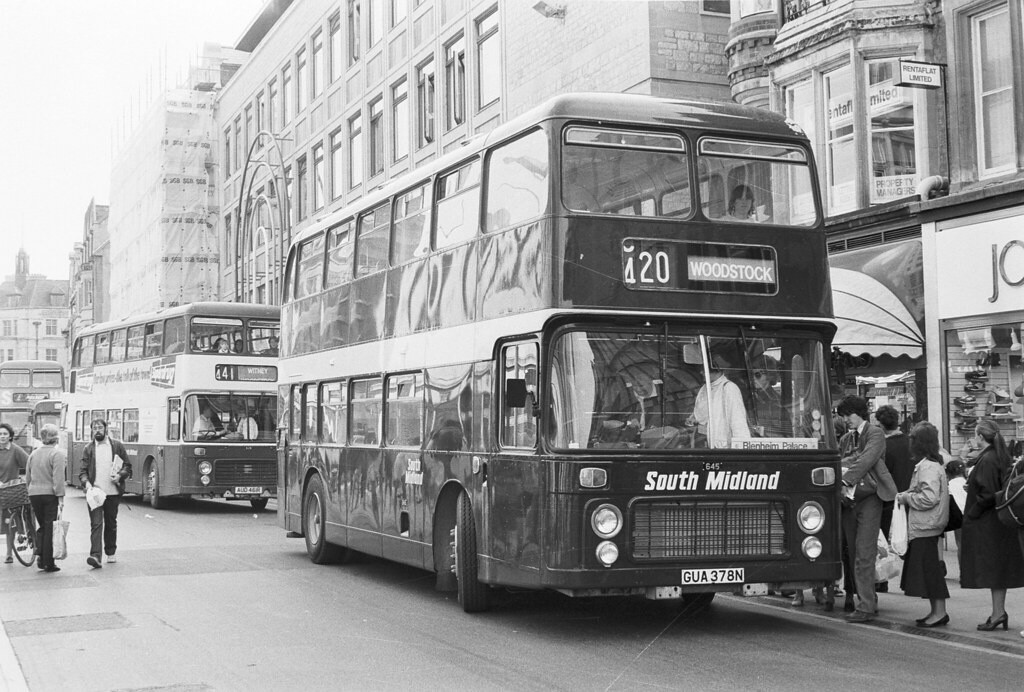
[0,480,39,568]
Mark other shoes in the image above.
[87,555,102,569]
[106,553,116,562]
[5,556,14,564]
[781,588,856,612]
[915,614,949,627]
[844,610,880,624]
[38,564,62,572]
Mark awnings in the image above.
[828,266,925,359]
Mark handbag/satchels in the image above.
[939,493,964,537]
[994,458,1024,531]
[840,446,878,510]
[53,505,70,560]
[886,492,909,557]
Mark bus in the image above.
[28,400,62,453]
[59,302,279,510]
[0,359,64,472]
[275,94,849,618]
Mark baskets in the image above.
[0,483,31,512]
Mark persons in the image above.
[25,423,66,572]
[612,363,673,444]
[174,331,279,355]
[791,394,1024,635]
[741,355,793,437]
[192,406,258,439]
[718,185,755,222]
[0,423,29,563]
[684,354,751,449]
[79,418,132,568]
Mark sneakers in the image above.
[964,384,987,393]
[964,370,989,383]
[976,353,1001,367]
[953,394,978,407]
[954,409,979,420]
[955,420,977,431]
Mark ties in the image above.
[853,431,859,447]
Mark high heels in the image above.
[977,612,1008,631]
